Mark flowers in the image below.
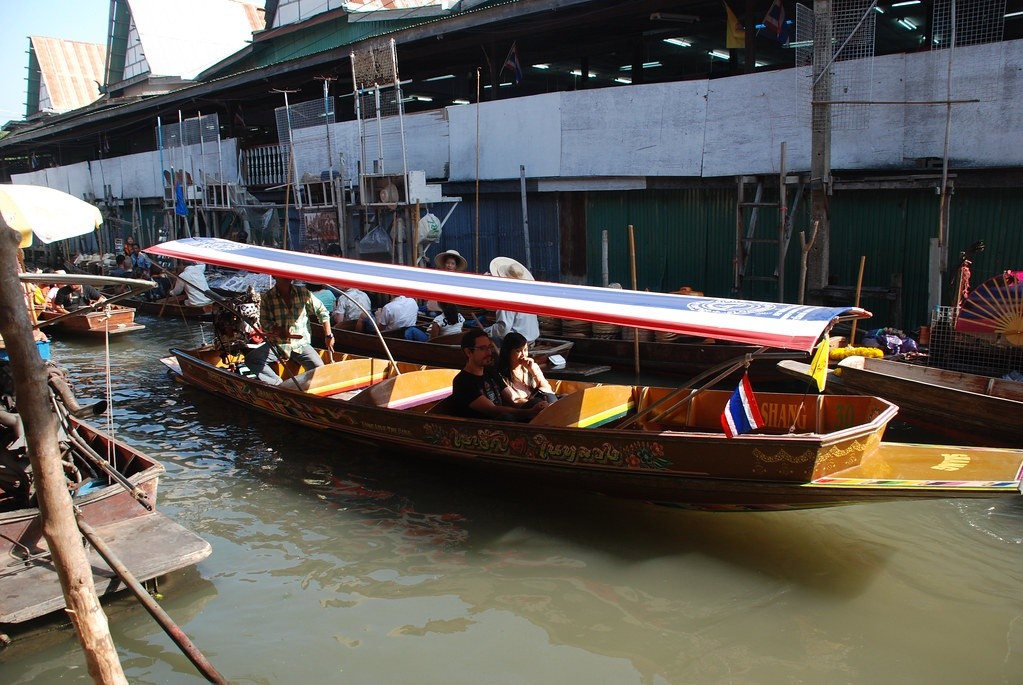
[830,347,884,377]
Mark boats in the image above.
[17,252,263,341]
[141,236,1023,516]
[0,409,215,629]
[775,355,1023,449]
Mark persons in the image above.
[167,257,215,307]
[405,300,466,342]
[244,275,335,386]
[24,266,111,315]
[305,282,337,321]
[355,295,419,334]
[111,235,152,281]
[427,250,467,311]
[70,248,97,263]
[327,244,342,258]
[482,257,541,349]
[335,286,372,324]
[451,329,571,423]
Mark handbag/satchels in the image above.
[358,208,392,254]
[417,209,441,244]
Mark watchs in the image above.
[324,333,334,339]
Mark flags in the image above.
[719,372,765,441]
[810,333,829,393]
[505,39,523,85]
[776,13,798,45]
[761,0,786,38]
[233,105,246,128]
[723,0,755,52]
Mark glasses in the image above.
[470,344,493,351]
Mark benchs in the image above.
[383,322,470,345]
[283,358,661,432]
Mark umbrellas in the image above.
[0,184,104,248]
[175,181,192,238]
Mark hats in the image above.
[434,250,467,271]
[149,261,162,272]
[54,270,66,275]
[490,257,535,281]
[380,185,398,210]
